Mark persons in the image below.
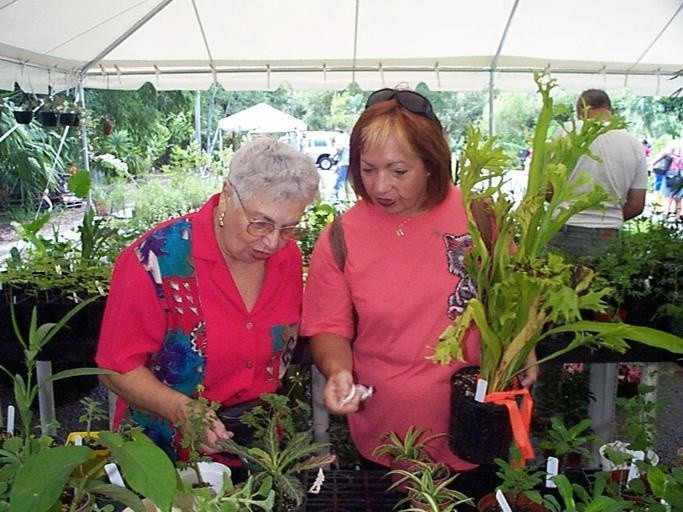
[327,134,350,201]
[299,87,540,474]
[544,89,646,263]
[91,137,320,485]
[639,137,682,222]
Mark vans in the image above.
[275,129,349,169]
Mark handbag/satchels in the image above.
[666,175,683,188]
[217,385,296,459]
[653,156,672,173]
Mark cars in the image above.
[494,141,530,170]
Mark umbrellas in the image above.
[217,101,307,135]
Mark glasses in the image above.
[220,176,310,241]
[364,88,432,119]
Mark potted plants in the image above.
[1,179,132,411]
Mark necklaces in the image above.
[380,208,415,238]
